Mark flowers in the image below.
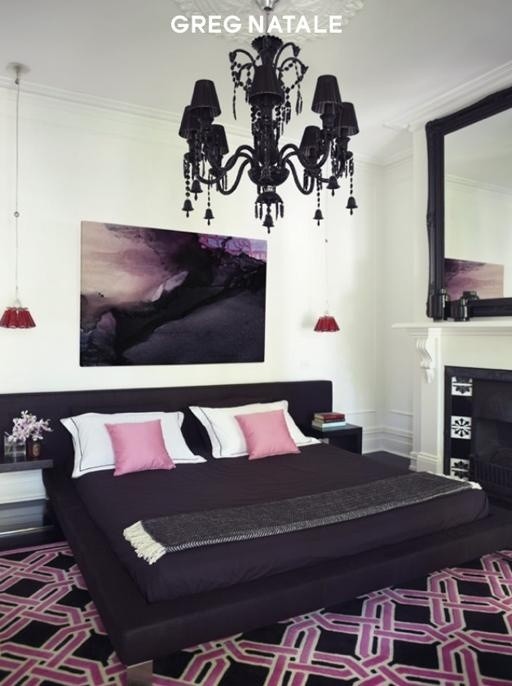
[8,411,51,440]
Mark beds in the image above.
[0,380,512,686]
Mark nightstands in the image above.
[306,423,363,455]
[0,453,56,550]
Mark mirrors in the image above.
[427,87,510,319]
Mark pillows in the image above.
[58,410,208,478]
[190,400,323,460]
[233,409,302,463]
[103,419,176,476]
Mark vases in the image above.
[29,439,41,458]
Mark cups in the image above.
[4,435,27,458]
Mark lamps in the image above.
[431,289,482,323]
[1,59,36,330]
[314,226,339,335]
[179,1,356,225]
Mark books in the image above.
[312,410,348,432]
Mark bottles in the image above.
[456,295,470,320]
[437,288,450,319]
